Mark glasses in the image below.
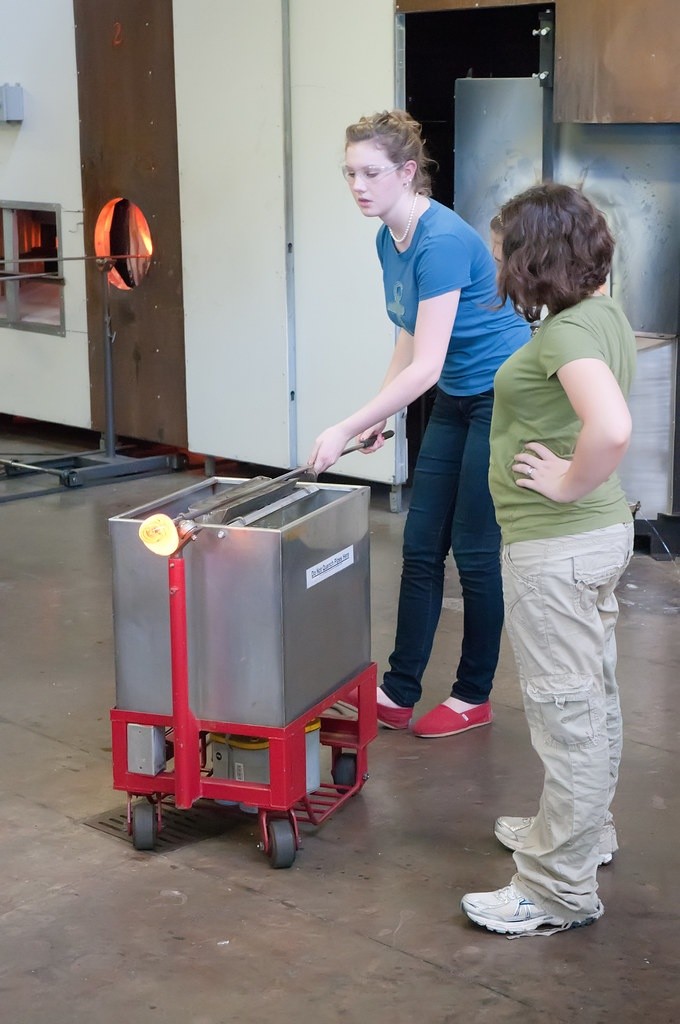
[341,162,400,184]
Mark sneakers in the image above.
[494,815,615,865]
[462,885,601,932]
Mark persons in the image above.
[303,107,533,739]
[455,180,639,939]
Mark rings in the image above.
[525,468,535,477]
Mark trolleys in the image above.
[105,475,377,870]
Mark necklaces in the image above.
[387,191,421,243]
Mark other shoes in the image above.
[336,685,413,730]
[413,702,492,738]
